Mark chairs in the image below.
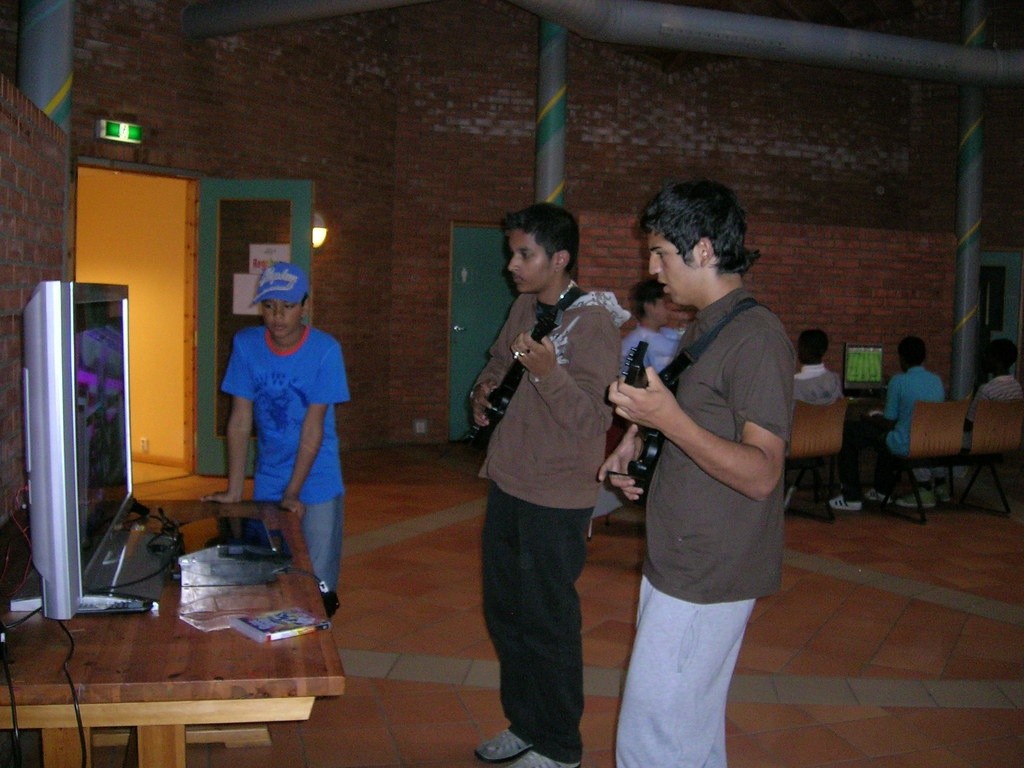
[878,399,967,525]
[971,398,1024,515]
[783,396,847,527]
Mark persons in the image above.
[897,338,1024,508]
[598,181,795,768]
[829,335,945,511]
[783,329,842,458]
[468,204,619,768]
[201,262,351,619]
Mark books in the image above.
[229,606,331,643]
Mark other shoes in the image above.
[323,594,341,619]
[785,480,796,511]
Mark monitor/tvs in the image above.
[9,279,174,622]
[843,343,885,396]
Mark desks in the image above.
[0,496,347,768]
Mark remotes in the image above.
[76,601,152,615]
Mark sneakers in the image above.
[897,487,936,507]
[865,489,894,504]
[936,484,952,502]
[829,496,862,511]
[474,728,583,767]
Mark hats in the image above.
[253,261,310,304]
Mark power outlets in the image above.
[410,419,428,437]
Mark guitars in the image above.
[462,313,558,455]
[622,339,664,502]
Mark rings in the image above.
[514,348,531,359]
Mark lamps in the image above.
[311,211,329,250]
